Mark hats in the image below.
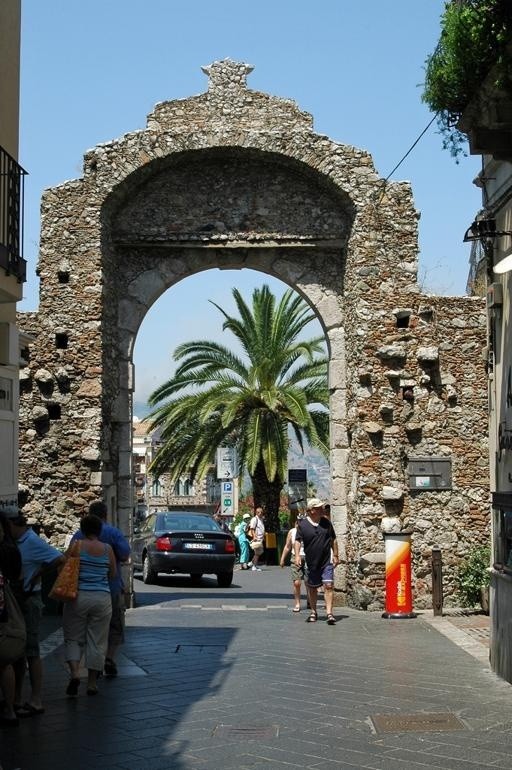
[243,514,251,519]
[307,497,326,510]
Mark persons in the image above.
[294,498,339,624]
[57,514,118,696]
[70,502,130,674]
[213,506,265,572]
[280,516,312,613]
[8,512,68,716]
[1,510,22,727]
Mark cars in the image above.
[131,510,235,588]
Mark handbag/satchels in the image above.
[50,556,80,599]
[2,583,26,659]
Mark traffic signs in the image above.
[217,448,238,478]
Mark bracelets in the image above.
[332,554,338,558]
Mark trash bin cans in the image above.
[40,562,63,617]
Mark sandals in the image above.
[15,659,117,722]
[308,612,317,621]
[327,613,336,622]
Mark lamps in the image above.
[492,246,512,274]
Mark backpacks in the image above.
[234,525,241,537]
